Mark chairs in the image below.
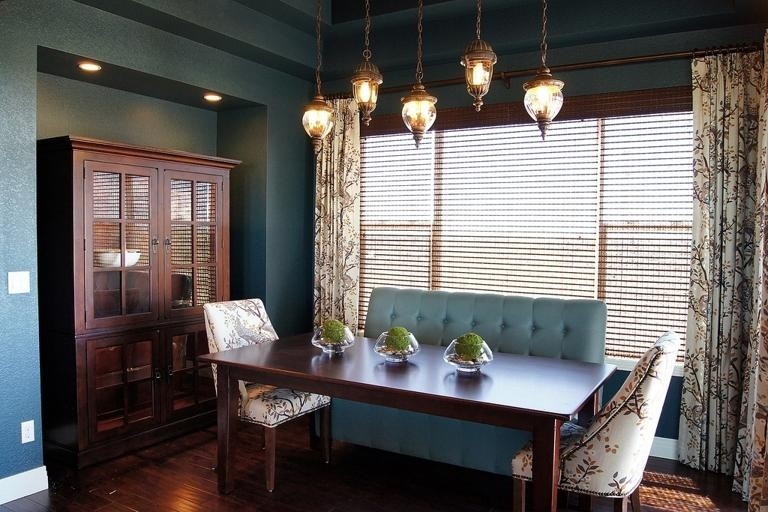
[511,330,684,511]
[202,299,331,493]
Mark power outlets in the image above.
[20,419,34,443]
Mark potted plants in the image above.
[374,326,421,362]
[313,319,355,357]
[443,333,493,377]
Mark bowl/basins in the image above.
[94,252,141,267]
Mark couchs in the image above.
[311,285,606,485]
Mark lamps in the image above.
[401,0,439,149]
[349,1,378,128]
[523,1,565,141]
[460,1,497,111]
[301,0,335,155]
